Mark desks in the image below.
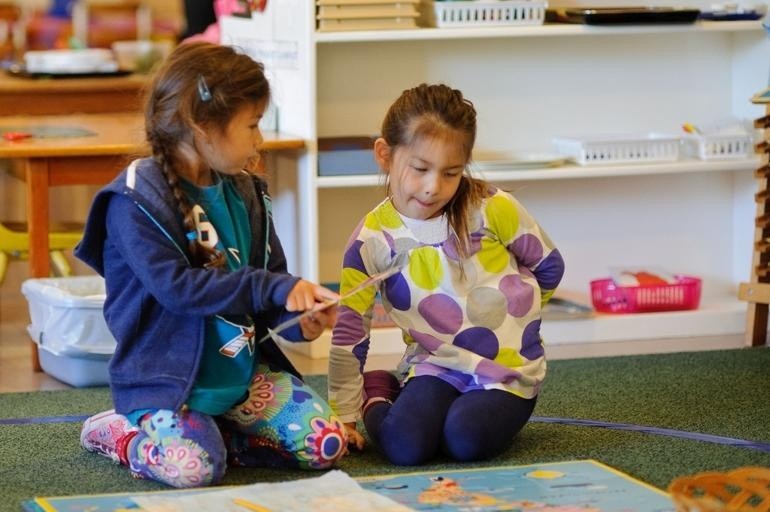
[2,70,155,122]
[0,111,308,373]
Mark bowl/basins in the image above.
[110,40,169,70]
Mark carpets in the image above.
[0,349,768,509]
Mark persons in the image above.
[74,40,349,488]
[327,84,565,464]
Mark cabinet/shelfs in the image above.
[314,19,769,355]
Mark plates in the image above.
[566,7,701,22]
[472,159,564,169]
[700,12,762,20]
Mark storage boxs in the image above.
[21,279,118,389]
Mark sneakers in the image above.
[80,409,137,464]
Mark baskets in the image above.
[567,130,679,164]
[590,276,703,314]
[685,134,753,161]
[425,1,547,28]
